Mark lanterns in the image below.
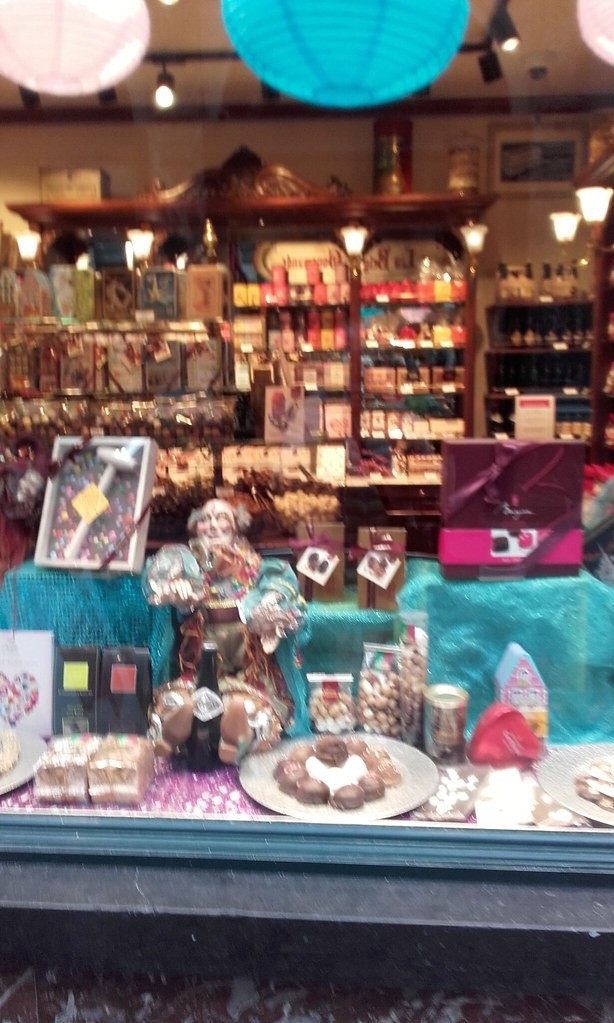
[0,0,151,98]
[222,0,468,109]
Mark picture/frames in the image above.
[492,128,584,194]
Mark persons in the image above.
[144,500,311,763]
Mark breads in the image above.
[33,733,159,806]
[577,754,614,814]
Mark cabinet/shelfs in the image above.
[4,193,502,554]
[592,152,614,464]
[483,301,593,442]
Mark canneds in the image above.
[233,259,352,307]
[422,684,470,767]
[267,305,349,352]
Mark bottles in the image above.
[446,144,475,196]
[511,313,593,349]
[494,258,536,302]
[184,641,222,772]
[488,403,514,440]
[491,365,592,396]
[605,312,614,344]
[555,405,592,440]
[380,132,406,195]
[603,413,614,450]
[568,258,581,300]
[539,261,567,304]
[269,308,347,353]
[602,362,614,398]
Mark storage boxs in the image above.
[440,435,583,580]
[39,169,110,201]
[36,433,158,575]
[356,526,407,613]
[296,521,345,602]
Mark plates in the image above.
[238,733,439,820]
[0,726,47,795]
[521,772,586,826]
[534,742,614,826]
[416,765,490,820]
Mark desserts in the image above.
[273,735,401,809]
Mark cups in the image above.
[423,683,467,761]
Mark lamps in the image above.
[494,13,521,53]
[479,51,503,84]
[155,63,176,107]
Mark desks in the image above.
[0,751,614,935]
